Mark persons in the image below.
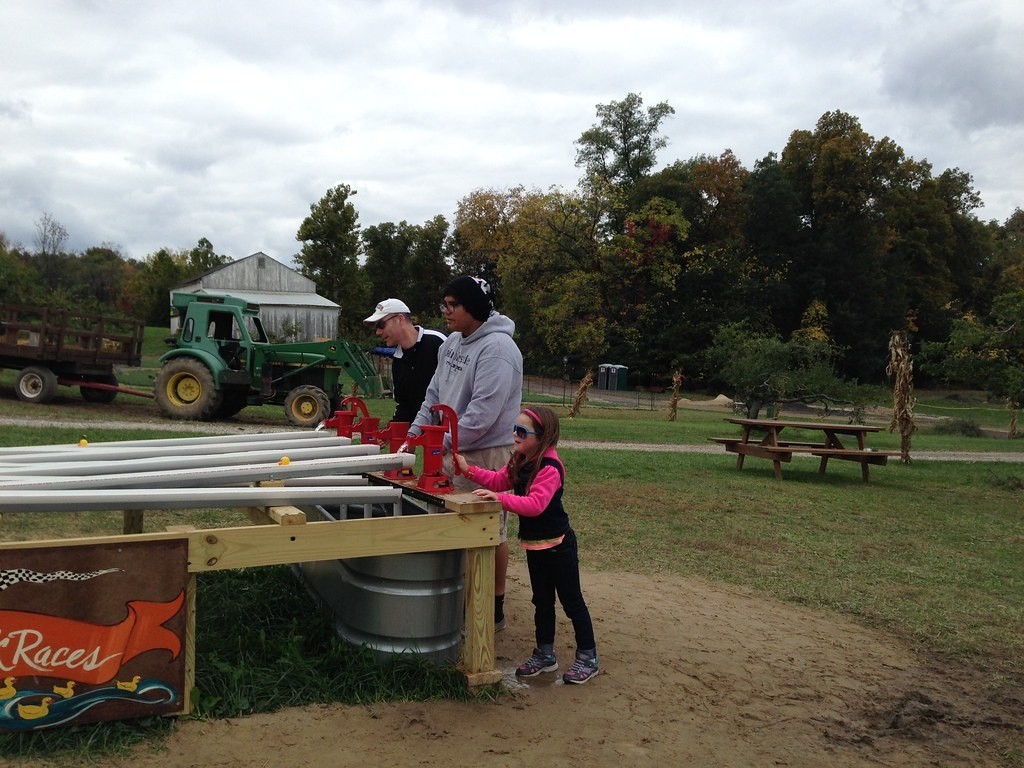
[452,405,600,685]
[362,297,449,450]
[404,276,525,633]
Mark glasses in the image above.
[376,315,399,329]
[513,424,539,439]
[442,301,461,308]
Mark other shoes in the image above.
[460,614,506,637]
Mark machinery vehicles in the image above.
[0,287,391,430]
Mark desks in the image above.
[722,418,886,483]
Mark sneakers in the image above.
[515,647,559,677]
[563,648,599,684]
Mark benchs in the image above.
[707,436,841,448]
[750,445,906,459]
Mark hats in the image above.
[363,298,411,328]
[442,276,493,321]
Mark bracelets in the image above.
[464,464,475,479]
